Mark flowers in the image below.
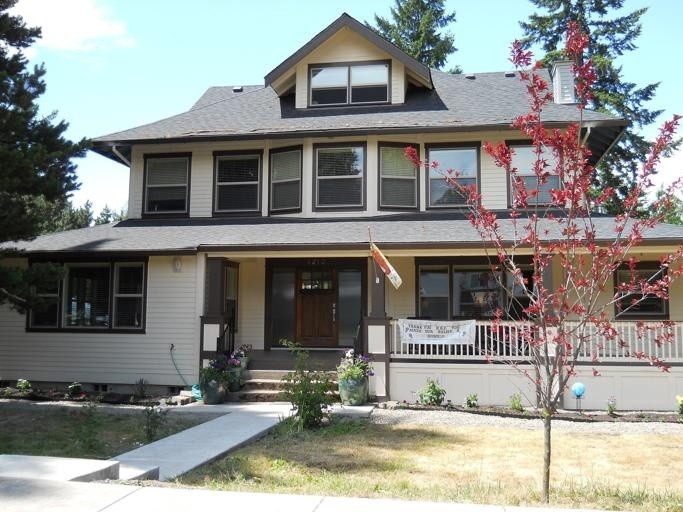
[335,348,374,381]
[197,343,250,388]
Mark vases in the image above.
[228,377,244,392]
[338,379,369,406]
[199,379,228,405]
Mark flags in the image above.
[370,241,402,291]
